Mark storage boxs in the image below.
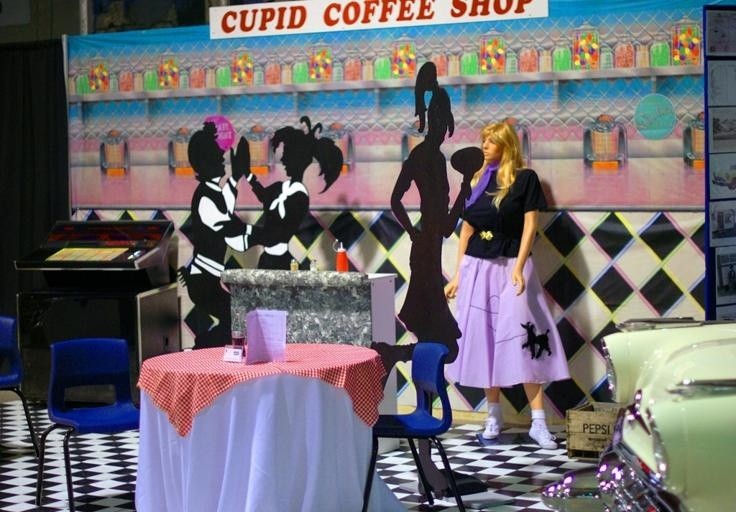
[565,400,627,459]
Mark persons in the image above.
[437,116,571,453]
[382,56,486,371]
[175,117,249,346]
[212,111,345,274]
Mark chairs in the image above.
[0,314,139,511]
[362,342,466,512]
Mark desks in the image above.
[220,270,398,457]
[139,344,380,511]
